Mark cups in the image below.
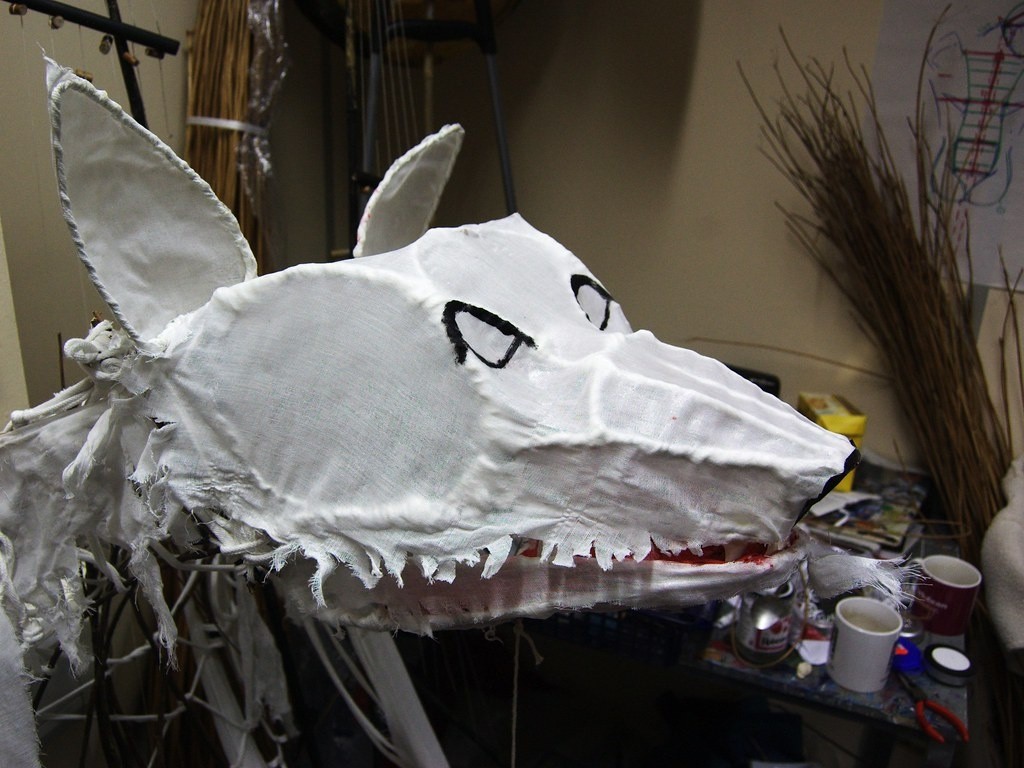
[730,577,797,668]
[823,596,904,693]
[910,555,984,638]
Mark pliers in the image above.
[896,669,973,744]
